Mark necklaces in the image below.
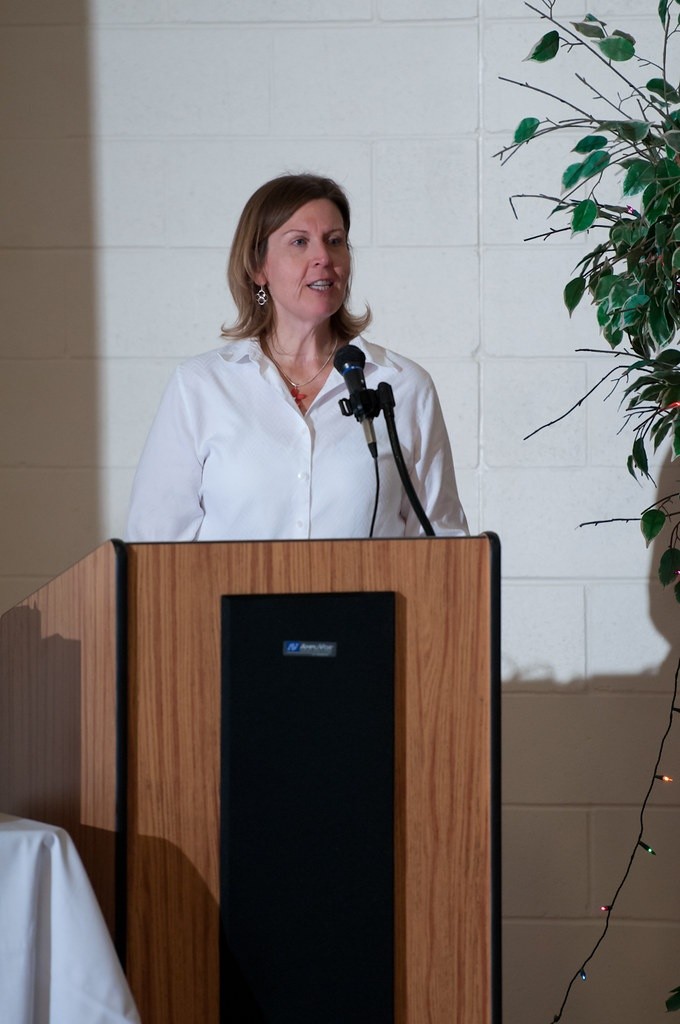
[262,335,339,408]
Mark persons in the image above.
[126,174,470,542]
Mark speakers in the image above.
[220,591,396,1024]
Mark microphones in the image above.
[332,344,379,459]
